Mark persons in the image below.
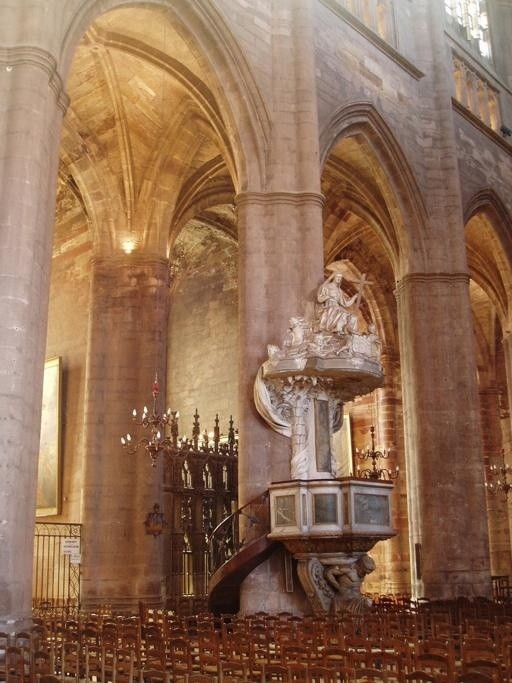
[316,271,358,335]
[326,556,376,607]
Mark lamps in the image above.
[142,503,167,539]
[118,373,180,470]
[350,425,399,482]
[485,449,512,501]
